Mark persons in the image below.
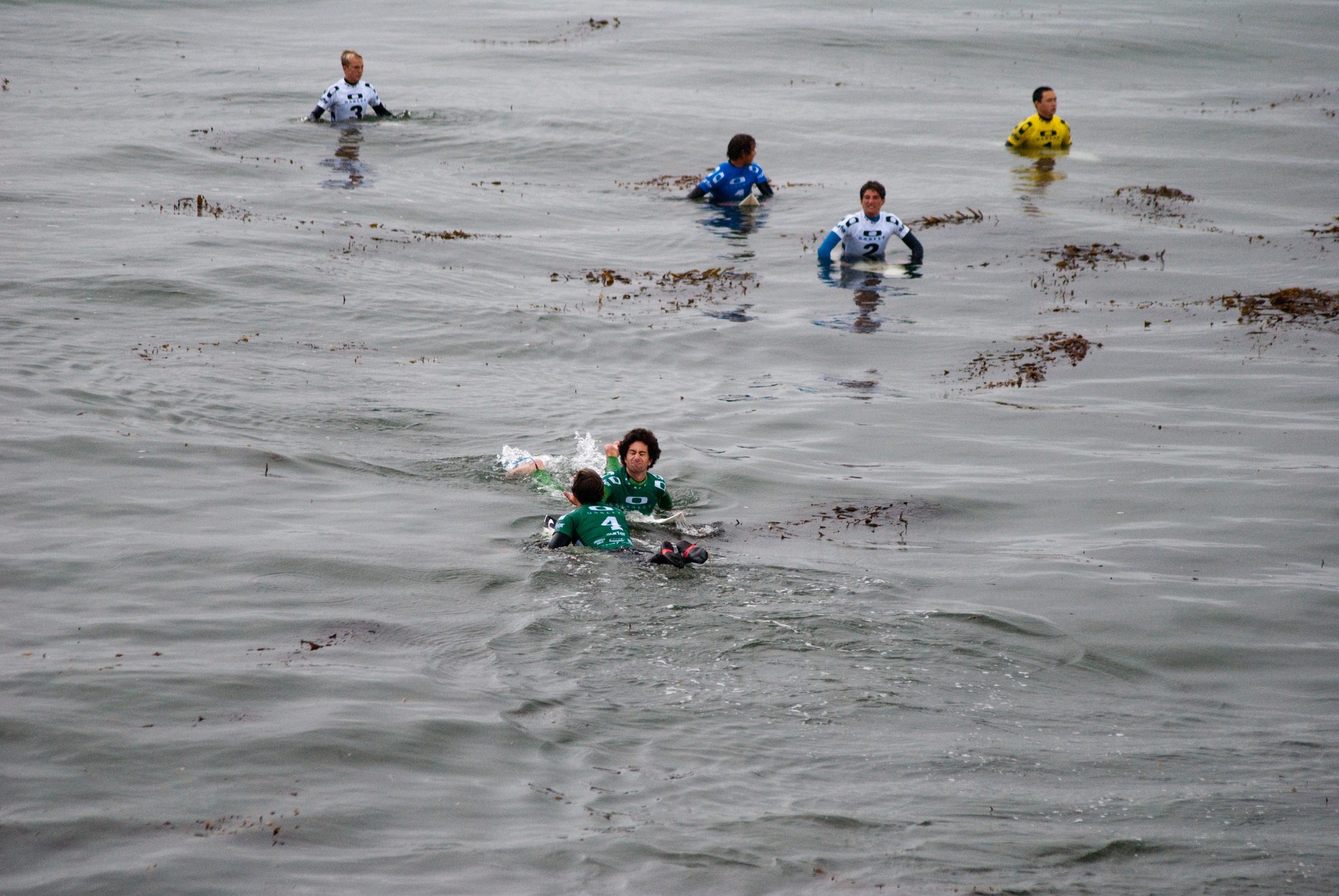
[688,133,772,196]
[306,49,393,116]
[818,181,922,261]
[507,430,672,515]
[1006,86,1072,148]
[549,467,709,567]
